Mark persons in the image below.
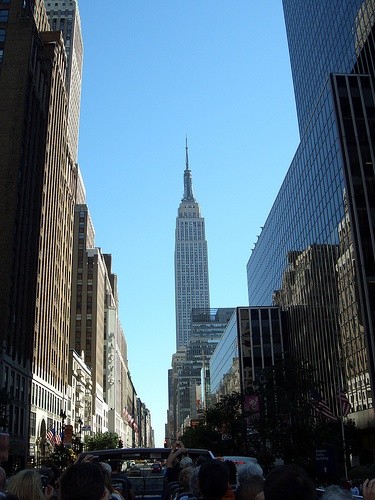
[0,440,375,500]
[8,468,53,500]
[61,462,105,500]
[199,461,230,500]
[264,462,318,500]
[0,467,8,500]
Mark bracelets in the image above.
[169,450,174,454]
[175,450,181,455]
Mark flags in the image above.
[53,433,63,445]
[46,429,55,442]
[336,385,351,417]
[310,390,337,421]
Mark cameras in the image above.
[87,455,99,464]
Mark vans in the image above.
[73,446,215,495]
